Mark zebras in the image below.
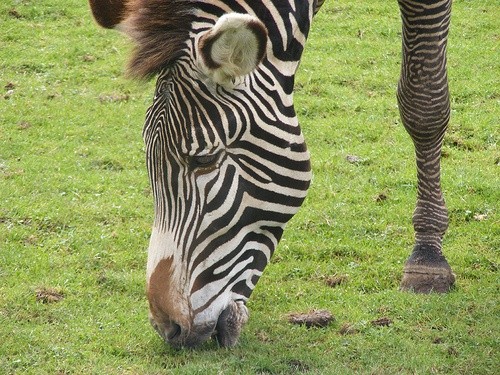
[87,0,456,351]
